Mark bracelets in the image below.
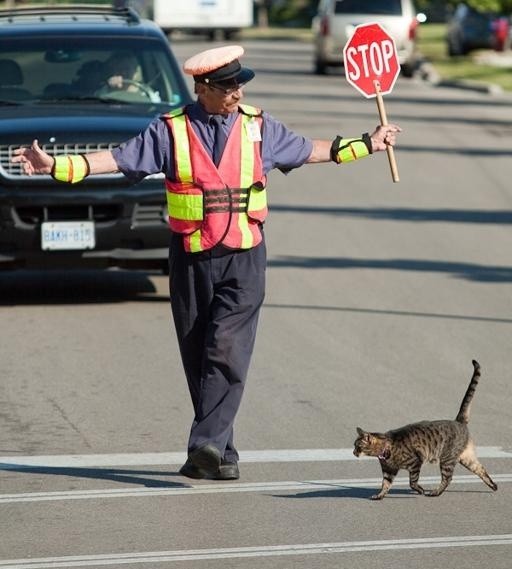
[330,129,373,166]
[51,153,90,185]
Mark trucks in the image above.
[129,1,256,43]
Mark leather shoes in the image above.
[208,462,239,481]
[178,446,221,479]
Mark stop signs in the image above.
[343,23,402,99]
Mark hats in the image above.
[180,44,256,90]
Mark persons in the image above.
[10,44,401,481]
[91,50,160,104]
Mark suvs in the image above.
[444,2,511,54]
[1,2,199,297]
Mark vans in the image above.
[308,1,424,77]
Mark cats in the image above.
[352,359,498,500]
